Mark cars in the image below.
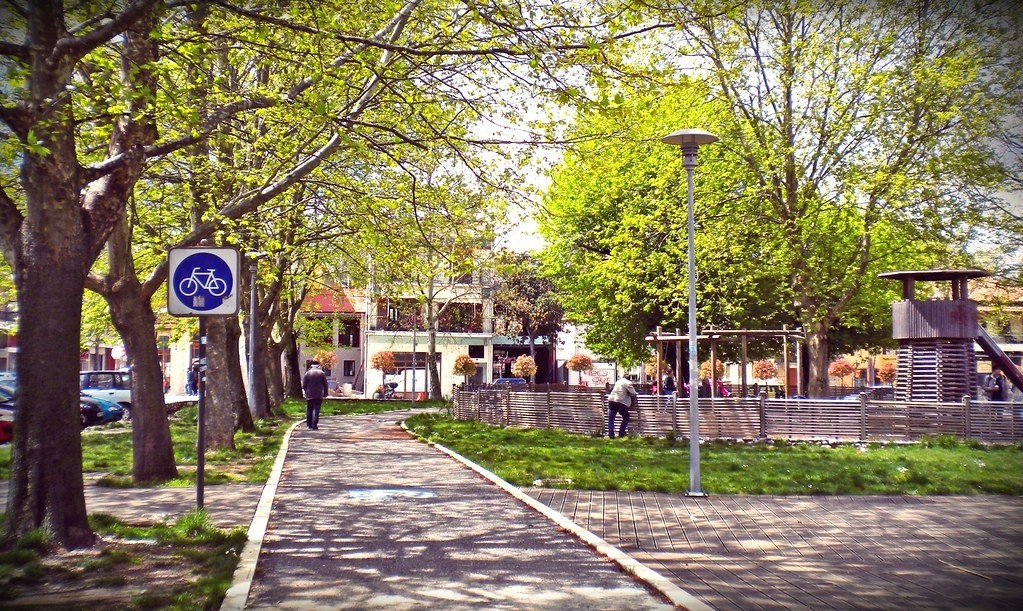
[78,370,132,421]
[92,398,124,421]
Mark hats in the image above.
[310,361,319,365]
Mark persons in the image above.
[185,367,198,396]
[702,374,711,398]
[608,374,636,439]
[302,361,328,430]
[1011,383,1023,434]
[651,381,657,398]
[754,383,759,397]
[774,386,785,398]
[982,365,1008,434]
[663,369,676,413]
[716,377,728,396]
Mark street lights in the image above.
[660,127,720,498]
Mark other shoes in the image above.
[313,426,318,430]
[309,425,312,428]
